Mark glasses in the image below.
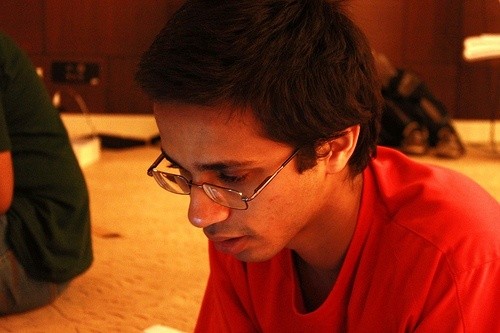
[146,139,308,212]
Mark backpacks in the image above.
[380,68,450,148]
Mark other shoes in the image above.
[436,127,466,158]
[401,123,430,153]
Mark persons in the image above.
[373,55,456,155]
[0,34,93,313]
[136,0,500,333]
